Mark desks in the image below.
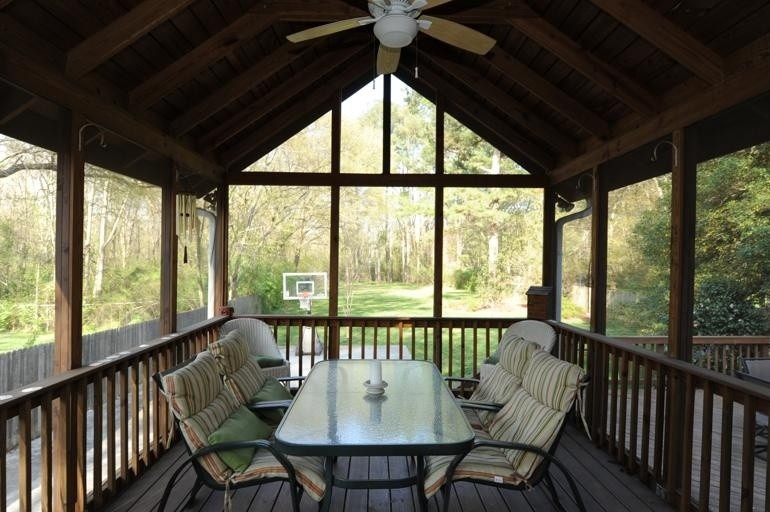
[273,356,475,512]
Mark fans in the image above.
[285,0,497,75]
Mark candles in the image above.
[370,358,382,385]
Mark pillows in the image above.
[208,404,273,471]
[249,375,293,420]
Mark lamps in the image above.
[373,13,418,48]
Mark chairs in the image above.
[424,350,588,511]
[156,349,327,512]
[220,315,293,394]
[444,334,545,430]
[741,356,770,461]
[481,321,557,361]
[205,329,307,431]
